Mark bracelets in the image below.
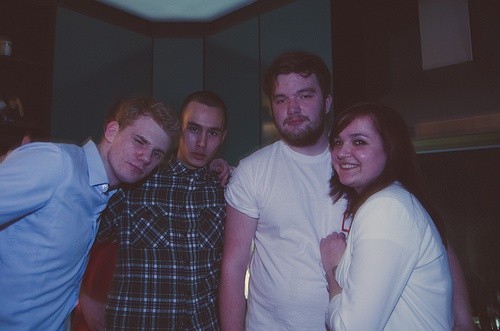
[325,265,339,277]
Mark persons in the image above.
[319,102,455,331]
[0,90,232,331]
[218,52,475,331]
